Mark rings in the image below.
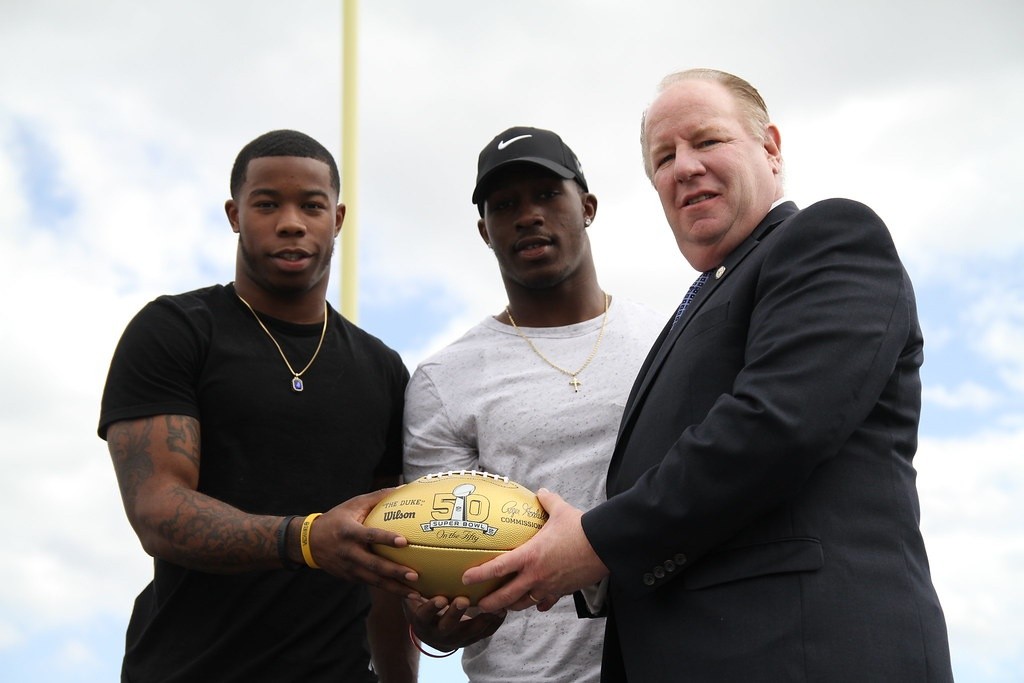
[527,592,543,605]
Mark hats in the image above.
[472,127,589,204]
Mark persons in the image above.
[461,68,954,683]
[398,127,664,683]
[96,130,420,683]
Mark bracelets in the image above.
[301,513,323,570]
[276,514,298,565]
[409,622,459,659]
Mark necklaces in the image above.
[233,284,328,391]
[504,291,609,393]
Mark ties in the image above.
[669,271,713,333]
